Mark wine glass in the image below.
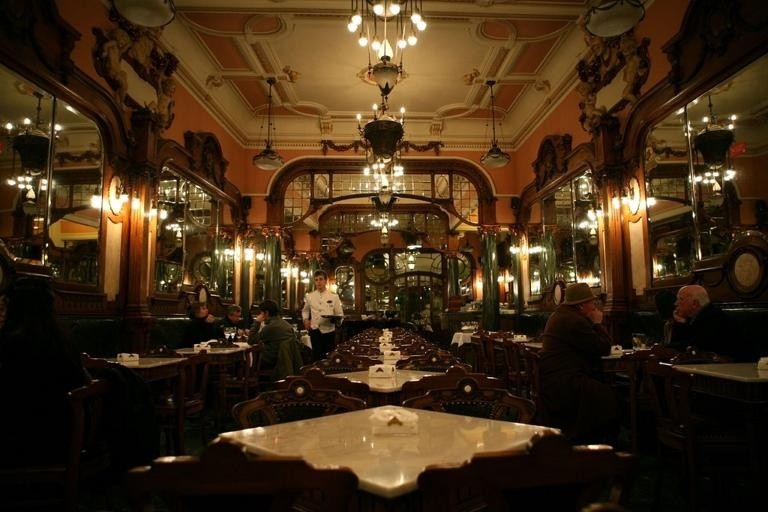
[224,326,236,343]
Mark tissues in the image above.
[116,328,622,436]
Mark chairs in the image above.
[1,301,767,511]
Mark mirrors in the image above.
[150,133,246,308]
[0,32,127,313]
[621,1,767,291]
[513,130,623,306]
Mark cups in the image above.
[632,333,646,352]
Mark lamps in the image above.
[581,1,647,38]
[104,1,178,31]
[347,0,427,271]
[682,84,739,196]
[1,78,71,204]
[157,168,206,240]
[245,240,314,283]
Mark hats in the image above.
[560,282,598,306]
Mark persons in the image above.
[664,283,740,366]
[539,282,615,404]
[185,271,345,384]
